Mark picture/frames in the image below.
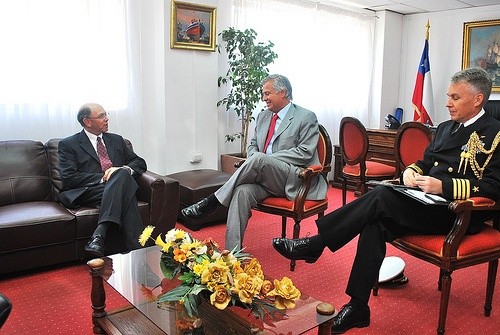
[462,19,500,93]
[171,0,217,52]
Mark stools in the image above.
[166,168,232,231]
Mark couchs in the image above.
[0,138,180,279]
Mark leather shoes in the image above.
[181,198,217,218]
[85,235,104,256]
[131,260,161,287]
[330,303,370,335]
[272,238,320,264]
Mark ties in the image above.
[453,124,465,138]
[263,114,278,153]
[97,137,112,173]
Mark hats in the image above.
[378,256,409,286]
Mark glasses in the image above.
[86,112,110,120]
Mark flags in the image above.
[411,39,435,126]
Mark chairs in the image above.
[393,122,434,180]
[252,124,332,272]
[373,197,500,335]
[339,117,396,205]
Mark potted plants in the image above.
[216,27,277,173]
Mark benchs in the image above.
[366,128,397,167]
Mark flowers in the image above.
[139,224,301,319]
[140,283,202,330]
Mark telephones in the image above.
[384,114,402,129]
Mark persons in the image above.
[272,68,500,335]
[59,103,160,286]
[182,74,327,255]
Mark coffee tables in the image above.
[87,245,338,335]
